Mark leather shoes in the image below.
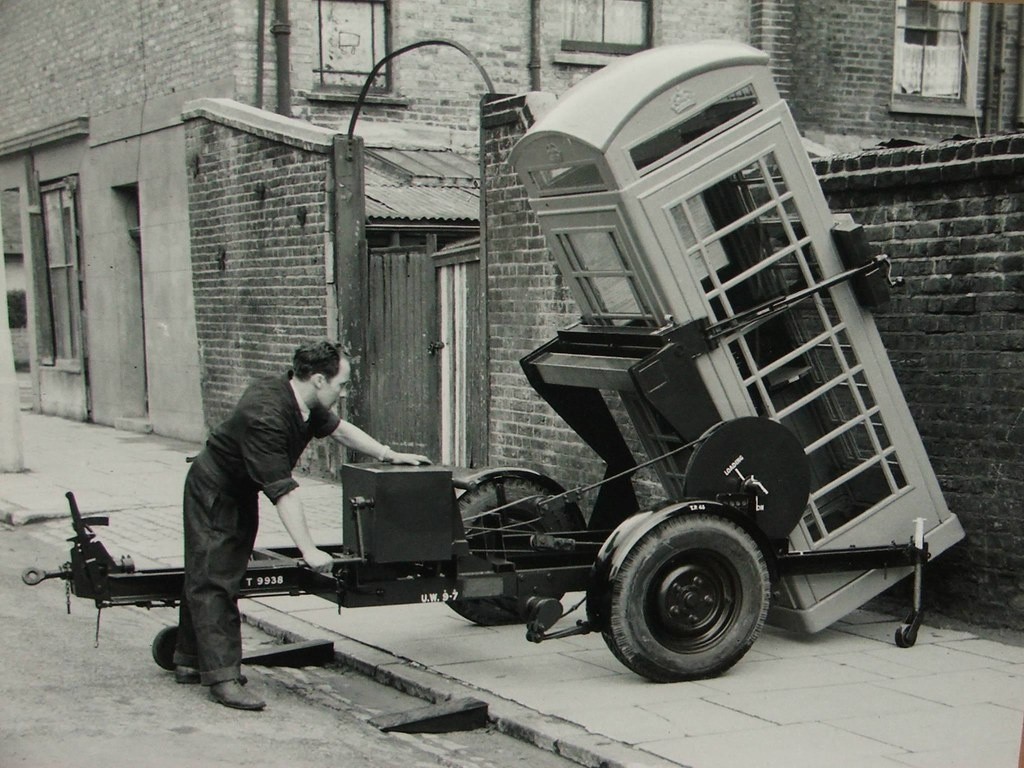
[209,679,267,709]
[175,665,248,686]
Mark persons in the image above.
[172,336,434,712]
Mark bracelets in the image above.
[379,444,391,462]
